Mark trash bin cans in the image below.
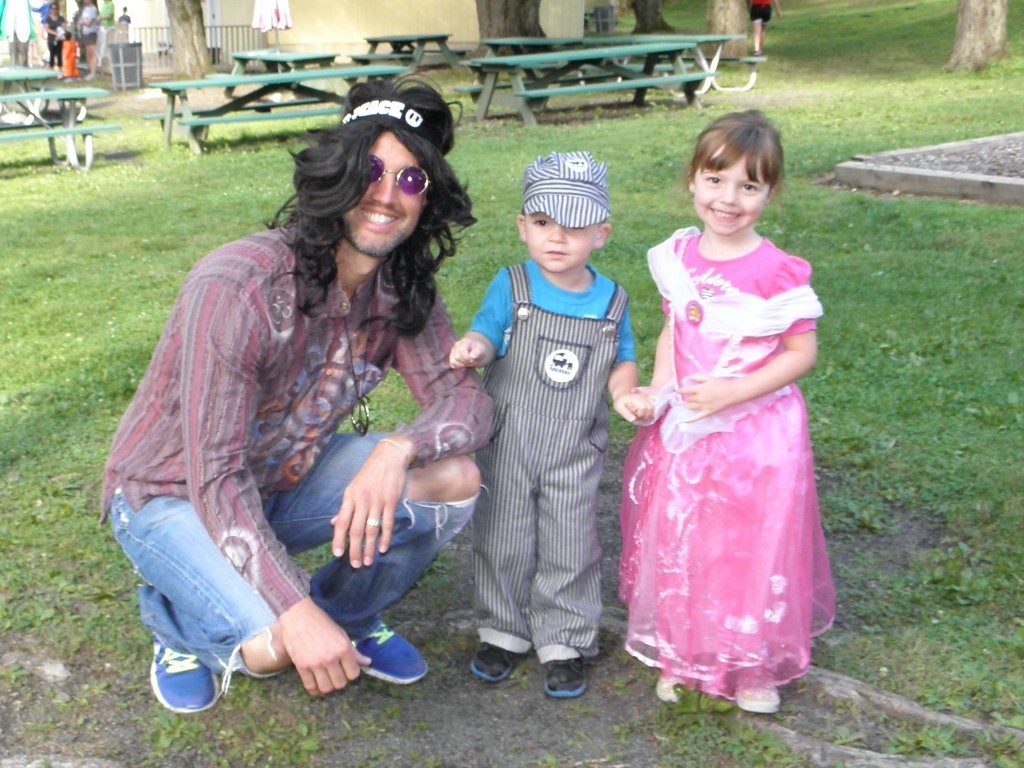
[110,43,144,90]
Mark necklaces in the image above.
[344,314,373,438]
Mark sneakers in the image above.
[543,659,587,697]
[150,633,217,713]
[469,640,513,682]
[345,618,427,684]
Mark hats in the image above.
[522,152,610,226]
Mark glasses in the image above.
[365,152,433,194]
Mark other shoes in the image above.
[734,683,781,714]
[656,676,684,702]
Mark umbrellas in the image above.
[0,0,36,66]
[250,0,293,53]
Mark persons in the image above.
[31,0,114,81]
[746,0,780,56]
[449,152,655,698]
[616,109,836,715]
[119,7,131,24]
[100,74,499,714]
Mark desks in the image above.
[479,36,580,80]
[470,43,703,129]
[0,88,110,113]
[0,69,56,93]
[583,34,739,94]
[147,65,408,153]
[234,46,339,72]
[365,35,454,52]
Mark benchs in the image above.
[0,117,121,169]
[454,50,767,100]
[408,49,470,57]
[351,53,414,64]
[141,97,345,129]
